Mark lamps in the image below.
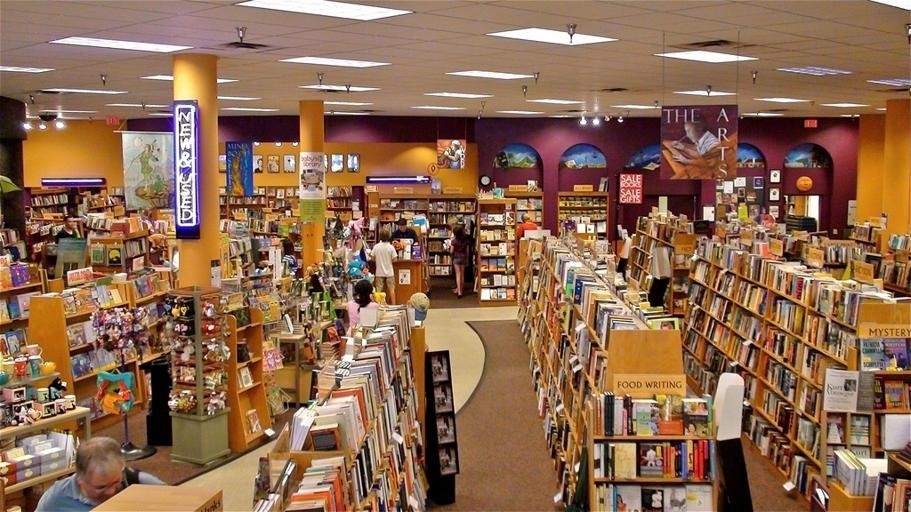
[575,109,629,129]
[24,117,67,134]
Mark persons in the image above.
[255,159,263,172]
[663,117,720,180]
[443,139,461,170]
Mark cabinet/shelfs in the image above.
[252,308,461,512]
[218,185,611,308]
[1,172,342,512]
[516,217,720,512]
[629,205,911,512]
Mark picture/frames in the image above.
[219,153,362,174]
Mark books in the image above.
[0,181,910,512]
